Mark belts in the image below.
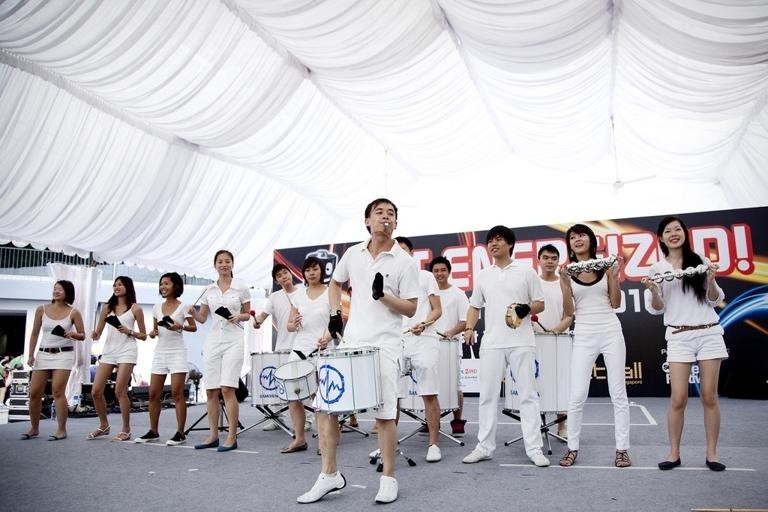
[663,322,724,334]
[37,343,76,355]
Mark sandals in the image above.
[87,423,109,441]
[19,428,42,442]
[48,430,68,443]
[110,429,131,442]
[615,449,631,467]
[559,449,579,466]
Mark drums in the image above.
[275,361,318,402]
[314,347,382,415]
[250,350,291,406]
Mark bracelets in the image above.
[465,327,472,332]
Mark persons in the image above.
[19,280,85,442]
[86,276,147,442]
[462,225,550,469]
[530,245,571,442]
[643,216,729,472]
[91,355,102,383]
[558,224,631,468]
[418,256,469,437]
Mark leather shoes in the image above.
[216,437,238,452]
[279,440,308,455]
[658,455,681,470]
[194,435,220,450]
[706,456,726,472]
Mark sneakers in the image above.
[557,426,573,443]
[371,474,402,504]
[415,419,469,440]
[260,415,363,433]
[134,429,159,445]
[296,470,347,504]
[527,451,550,467]
[369,442,399,459]
[165,431,186,446]
[426,442,442,463]
[462,444,495,463]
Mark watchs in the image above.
[128,330,134,336]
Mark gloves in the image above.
[327,309,344,340]
[515,303,531,319]
[372,272,385,301]
